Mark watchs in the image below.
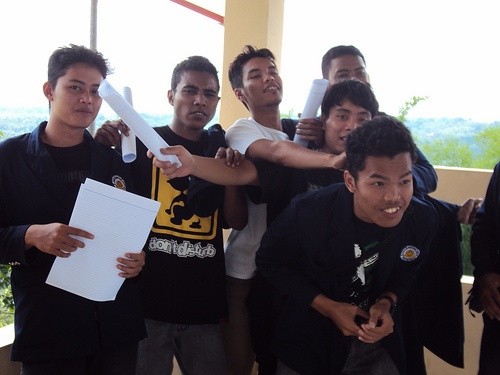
[379,295,397,315]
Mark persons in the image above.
[224,45,348,375]
[0,43,162,375]
[281,46,439,195]
[256,115,440,375]
[469,161,500,375]
[146,79,483,375]
[95,57,249,375]
